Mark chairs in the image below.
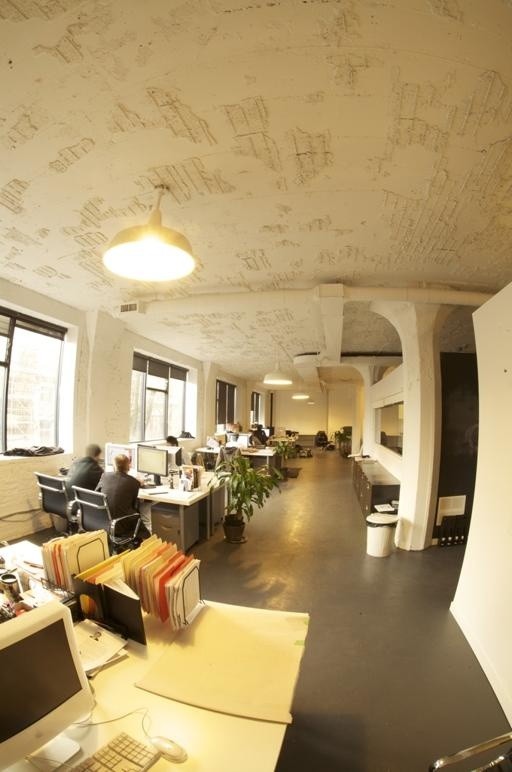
[33,471,141,556]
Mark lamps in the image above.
[102,185,197,285]
[262,346,310,400]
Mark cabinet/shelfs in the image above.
[352,459,400,520]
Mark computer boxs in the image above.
[139,502,160,535]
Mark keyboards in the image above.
[70,731,161,772]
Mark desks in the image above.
[99,467,232,556]
[195,435,296,477]
[0,540,313,771]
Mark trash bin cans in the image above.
[365,512,399,558]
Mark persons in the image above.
[90,632,101,642]
[250,425,269,447]
[166,436,194,466]
[65,444,105,501]
[95,453,150,541]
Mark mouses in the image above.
[152,736,187,761]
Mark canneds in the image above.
[0,573,21,604]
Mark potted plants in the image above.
[272,439,299,482]
[205,453,285,541]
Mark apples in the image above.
[79,594,96,619]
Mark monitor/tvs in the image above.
[105,444,182,486]
[214,426,274,450]
[0,599,94,772]
